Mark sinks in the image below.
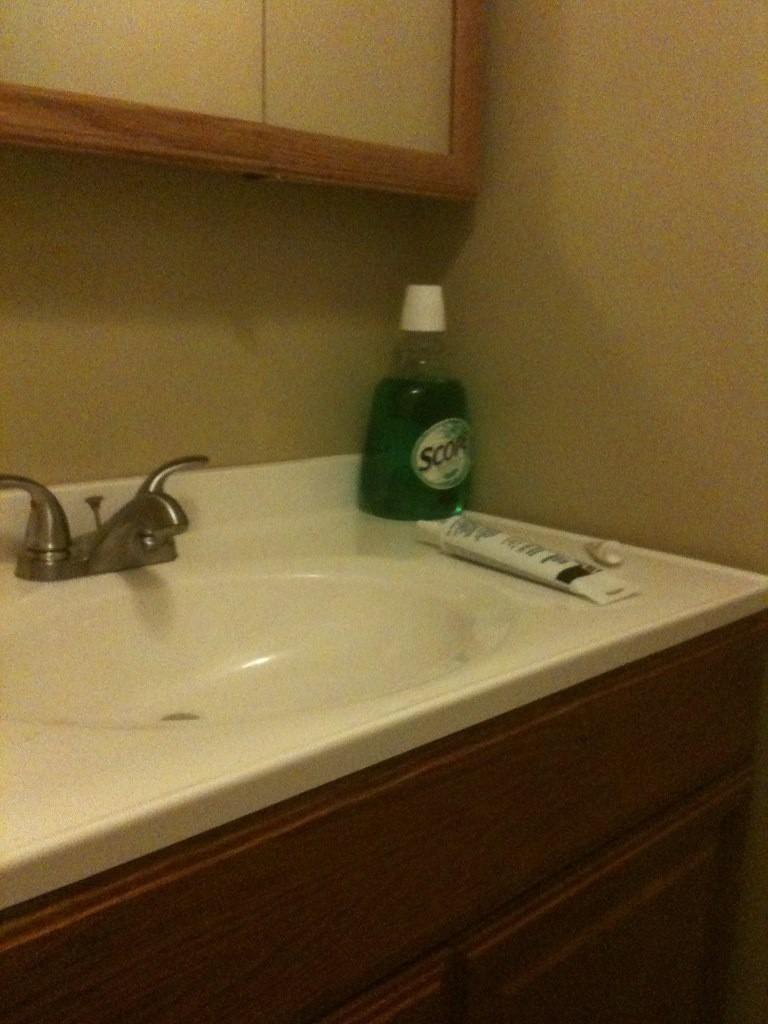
[0,558,515,742]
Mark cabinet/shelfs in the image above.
[0,613,768,1024]
[0,0,490,204]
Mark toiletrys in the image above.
[355,281,484,522]
[410,516,642,606]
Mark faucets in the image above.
[88,490,191,558]
